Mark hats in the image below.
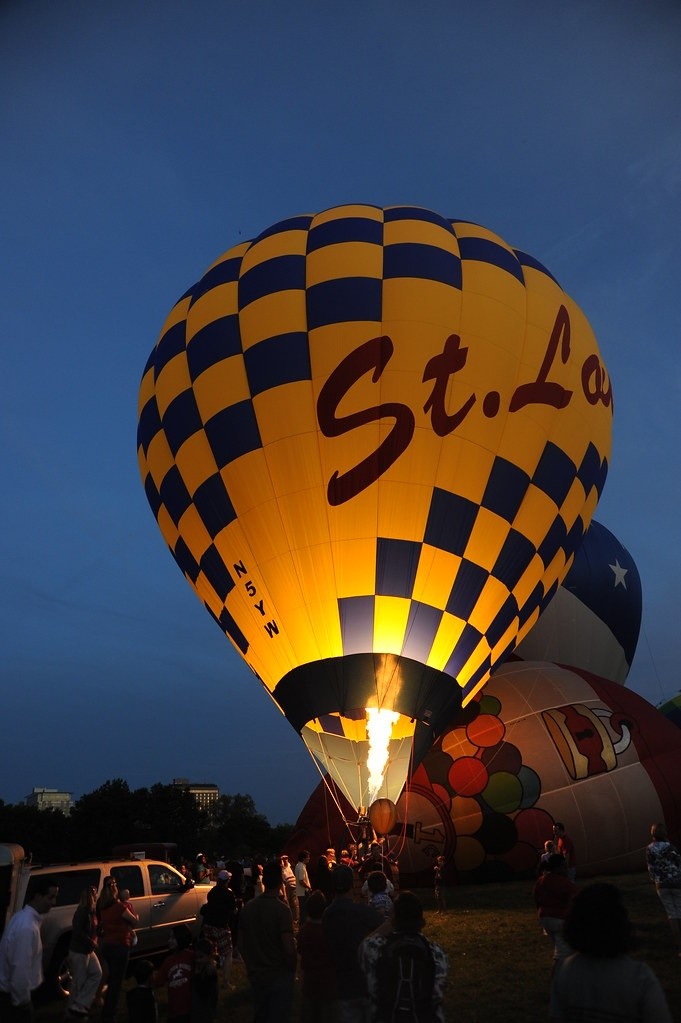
[218,869,232,880]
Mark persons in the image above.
[0,840,449,1023]
[550,883,672,1023]
[646,823,681,957]
[534,823,580,977]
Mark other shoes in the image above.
[672,951,681,957]
[224,984,236,991]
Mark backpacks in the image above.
[373,934,436,1023]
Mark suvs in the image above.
[17,856,236,1003]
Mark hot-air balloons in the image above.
[136,203,612,891]
[274,519,680,890]
